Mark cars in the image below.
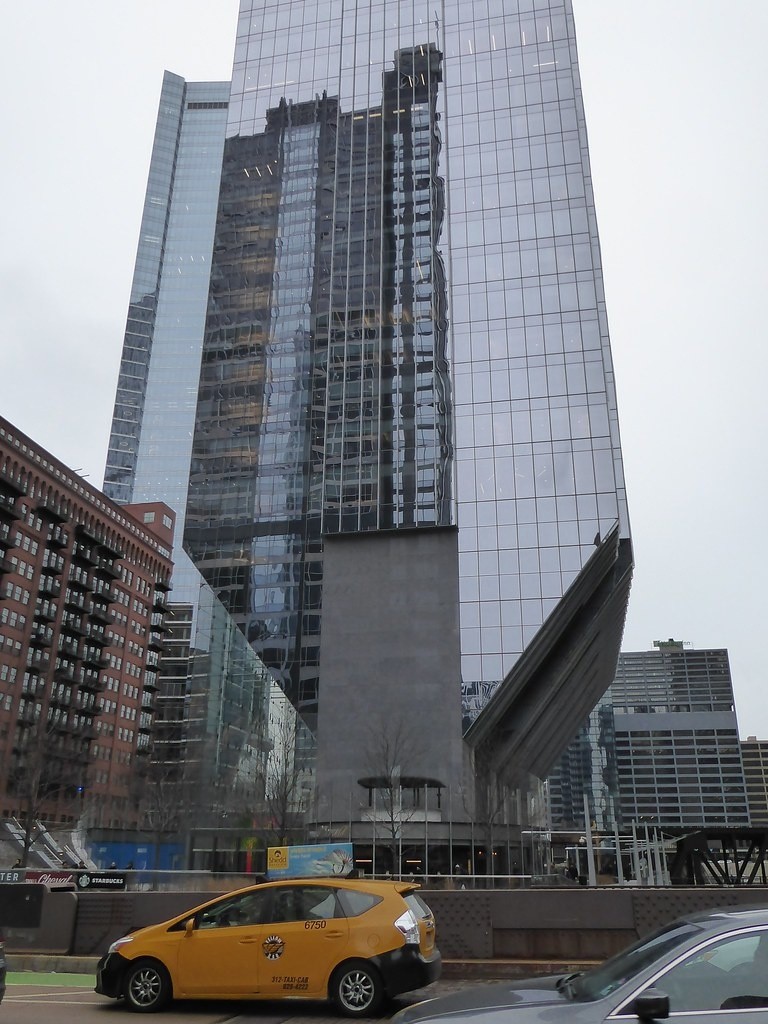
[93,879,441,1020]
[391,901,768,1024]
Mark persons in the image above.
[125,862,135,869]
[12,858,21,869]
[62,862,69,868]
[79,861,87,869]
[72,863,78,868]
[564,864,578,882]
[110,861,119,869]
